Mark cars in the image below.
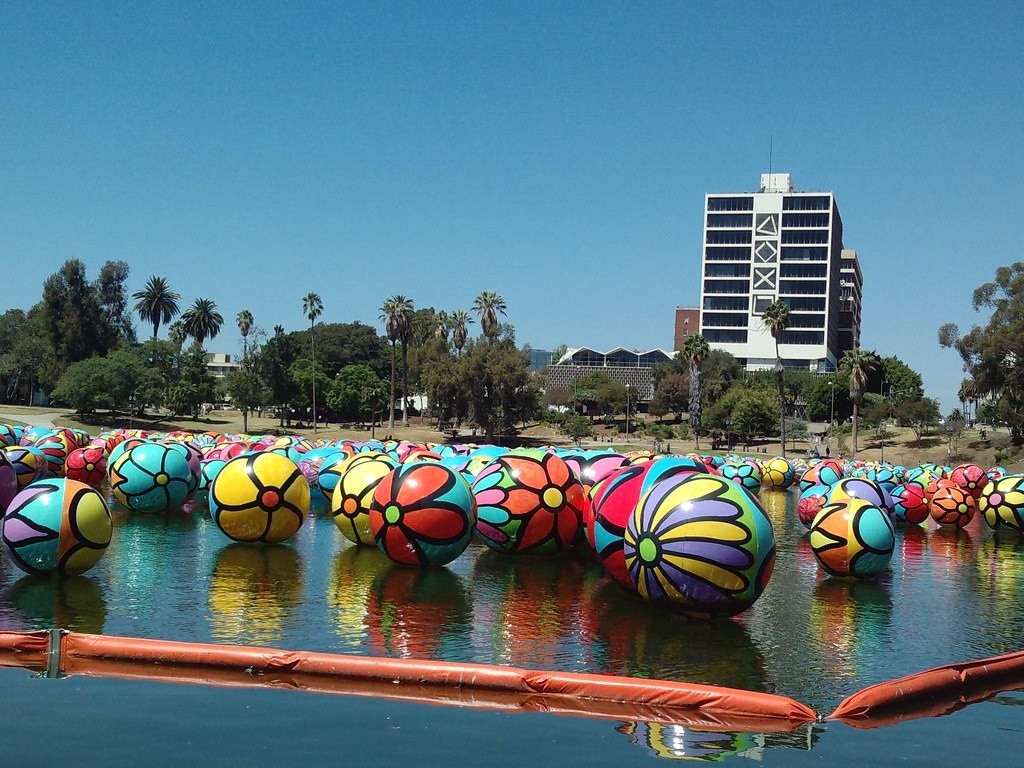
[975,423,993,431]
[219,403,234,411]
[263,405,275,413]
[214,404,221,410]
[198,403,215,410]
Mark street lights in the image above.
[572,375,577,413]
[625,382,630,443]
[828,382,835,439]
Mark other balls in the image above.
[0,422,1024,621]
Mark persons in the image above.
[815,434,824,446]
[826,446,830,457]
[814,446,820,457]
